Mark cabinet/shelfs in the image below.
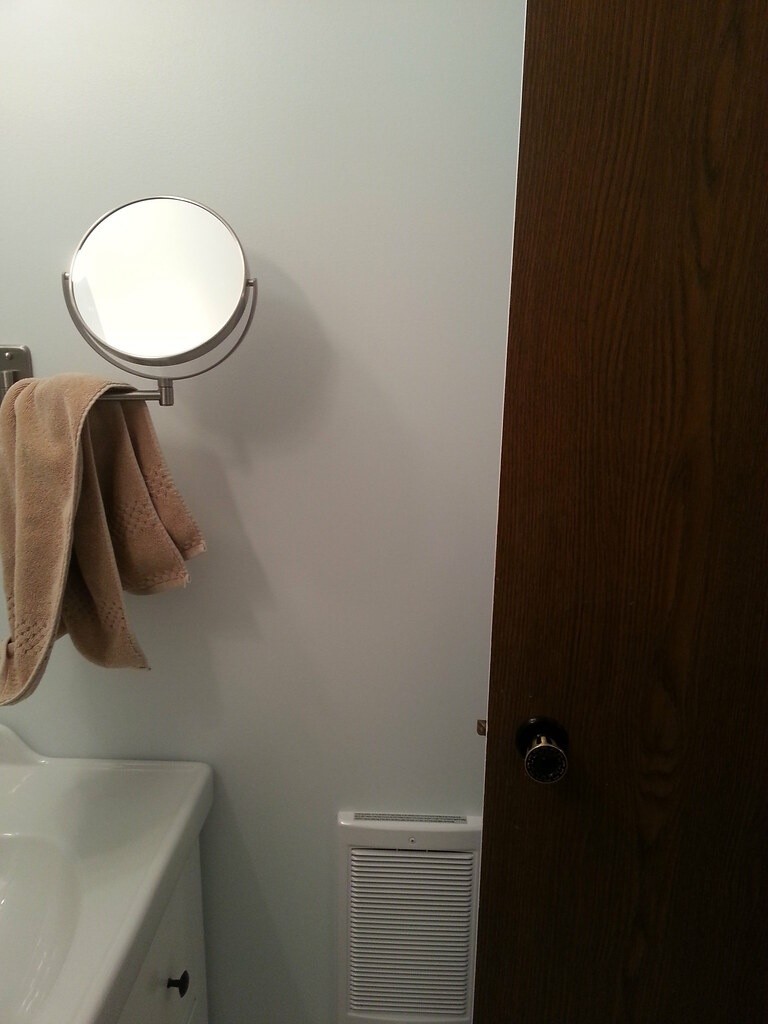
[116,838,209,1024]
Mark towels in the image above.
[0,374,207,705]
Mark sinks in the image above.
[0,831,83,1024]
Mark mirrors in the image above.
[61,195,257,381]
